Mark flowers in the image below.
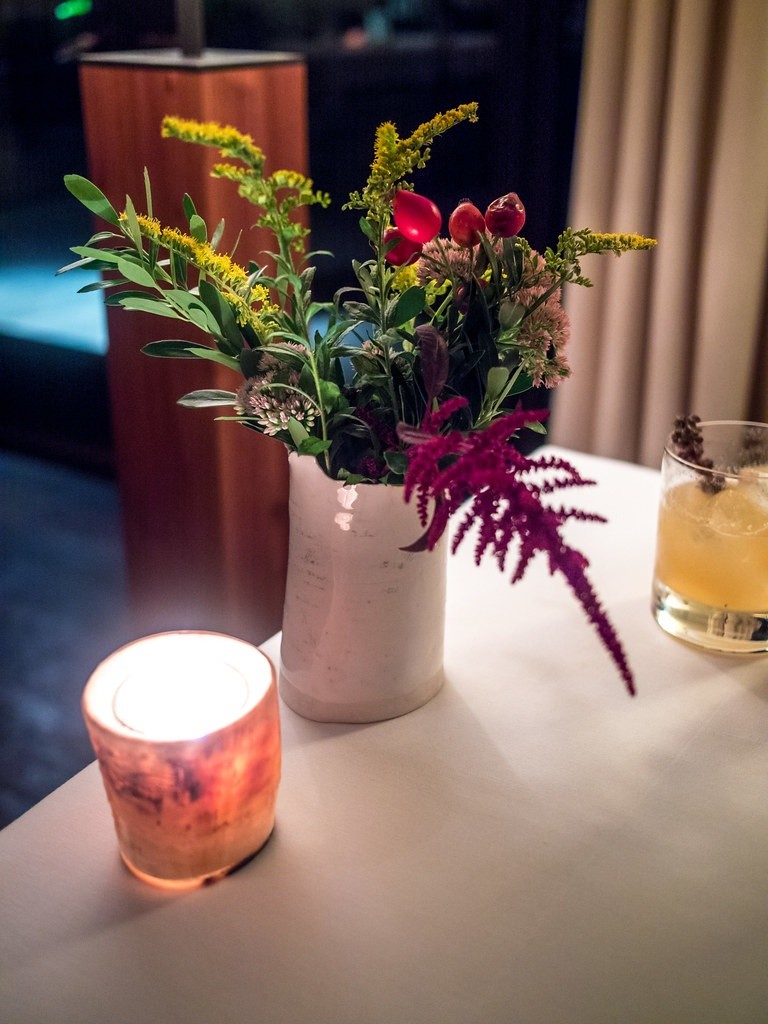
[58,100,655,697]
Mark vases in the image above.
[280,443,452,723]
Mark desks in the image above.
[1,443,768,1024]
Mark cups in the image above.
[651,421,768,657]
[82,630,282,890]
